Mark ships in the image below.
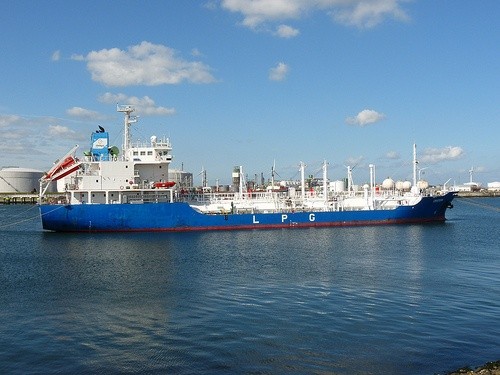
[39,103,458,234]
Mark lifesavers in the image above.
[153,180,177,188]
